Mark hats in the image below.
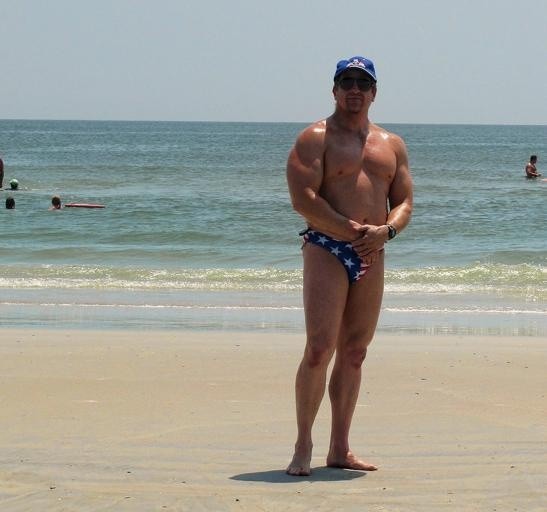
[334,55,377,81]
[9,179,18,188]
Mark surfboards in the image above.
[65,204,106,208]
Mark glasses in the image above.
[338,78,373,91]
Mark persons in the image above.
[525,156,542,178]
[0,158,4,188]
[7,179,22,190]
[48,196,63,211]
[5,197,14,209]
[287,58,413,476]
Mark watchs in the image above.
[385,224,396,239]
